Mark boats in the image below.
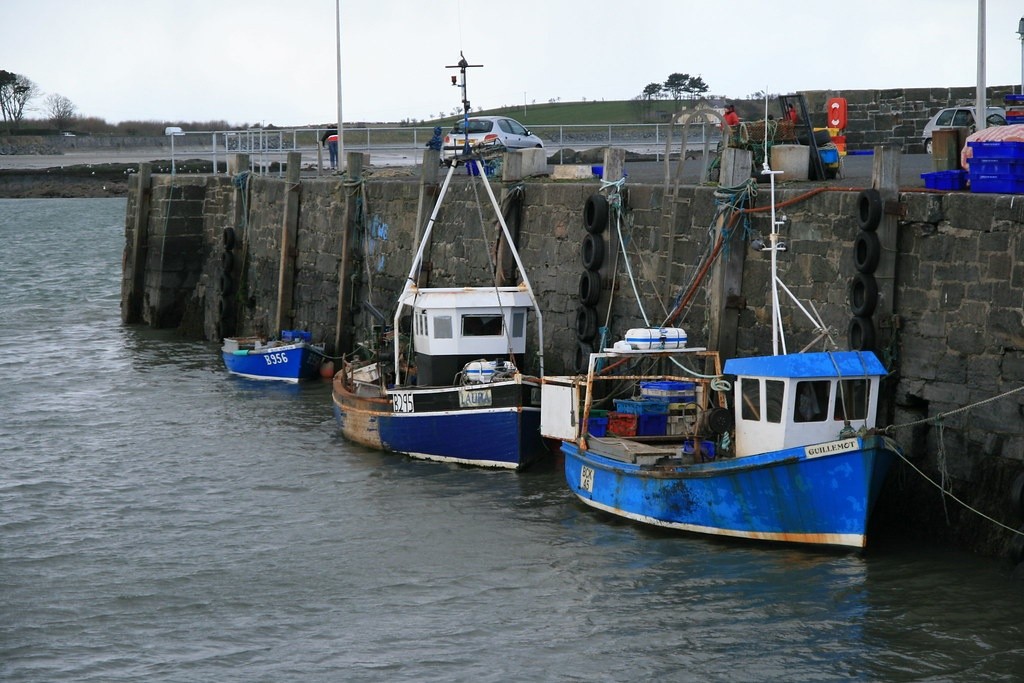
[331,49,564,472]
[539,86,889,550]
[221,329,326,385]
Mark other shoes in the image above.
[330,166,335,171]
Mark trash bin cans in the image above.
[931,127,970,171]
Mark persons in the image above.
[786,103,797,123]
[322,123,338,169]
[425,126,443,166]
[719,104,740,131]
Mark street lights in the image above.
[1014,16,1024,96]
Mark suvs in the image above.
[921,105,1006,154]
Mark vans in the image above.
[165,126,186,136]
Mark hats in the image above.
[724,105,734,109]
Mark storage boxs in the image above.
[613,399,669,414]
[684,440,715,460]
[668,410,696,416]
[579,418,608,437]
[640,388,695,396]
[579,409,607,419]
[640,381,695,390]
[666,416,696,435]
[282,330,310,343]
[669,403,696,409]
[607,411,638,436]
[466,160,483,176]
[920,170,968,191]
[966,158,1024,175]
[966,174,1024,194]
[642,395,696,403]
[967,142,1024,158]
[347,362,381,382]
[637,413,668,436]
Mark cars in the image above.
[442,114,543,168]
[61,131,76,137]
